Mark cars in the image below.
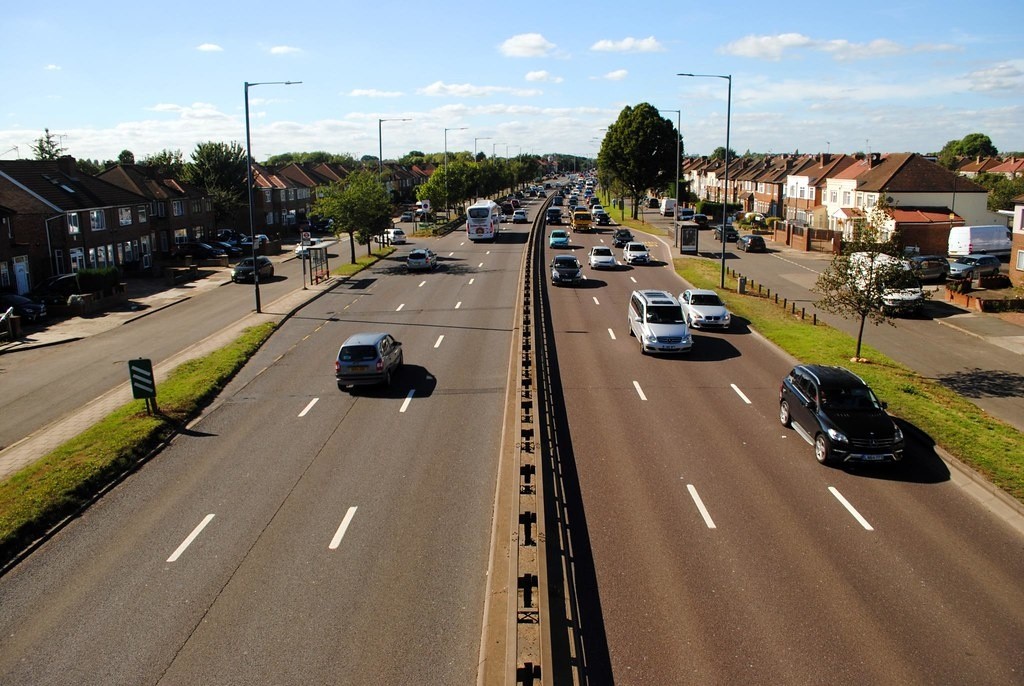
[548,230,570,249]
[500,173,611,225]
[949,254,1000,280]
[299,218,334,235]
[588,246,616,269]
[677,208,709,229]
[401,208,444,223]
[0,295,47,326]
[295,238,323,259]
[172,229,269,266]
[679,291,731,330]
[232,257,274,283]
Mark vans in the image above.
[846,252,925,317]
[645,198,659,208]
[659,198,675,216]
[947,225,1013,258]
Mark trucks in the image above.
[569,205,592,235]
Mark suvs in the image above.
[714,224,738,242]
[612,228,649,265]
[548,255,583,287]
[779,365,904,467]
[335,333,403,390]
[911,254,951,285]
[374,228,407,246]
[407,248,437,272]
[736,235,763,253]
[628,290,693,355]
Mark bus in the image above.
[466,200,501,241]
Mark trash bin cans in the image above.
[189,265,198,277]
[221,255,229,266]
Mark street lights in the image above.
[378,118,412,247]
[444,128,541,220]
[677,73,732,288]
[244,81,303,313]
